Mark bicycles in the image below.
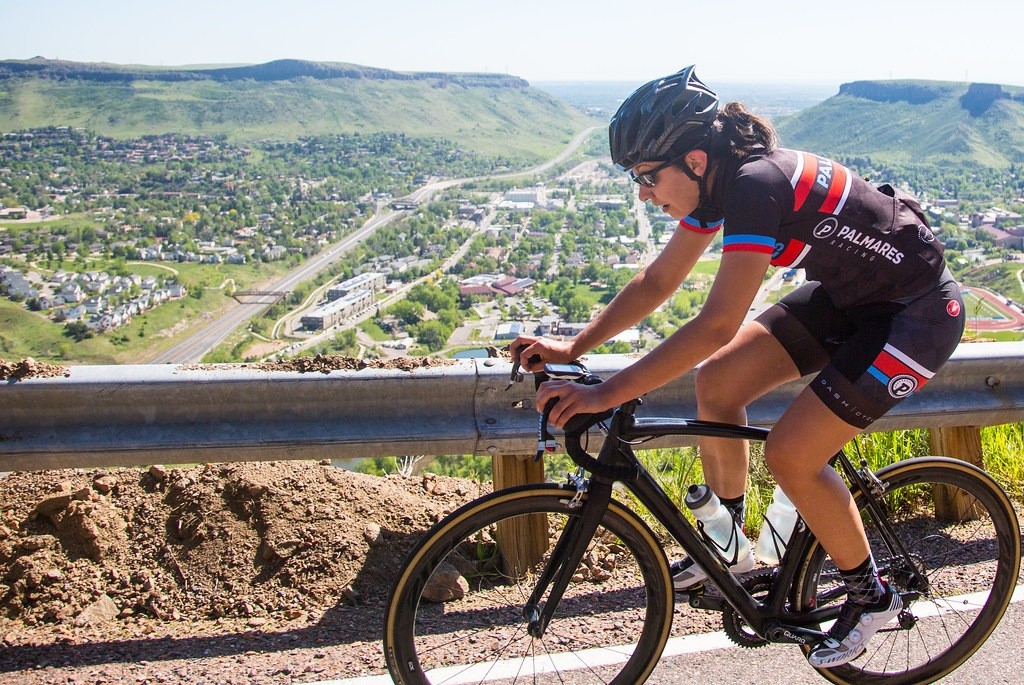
[384,343,1022,685]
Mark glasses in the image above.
[629,135,711,188]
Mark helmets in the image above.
[609,64,719,172]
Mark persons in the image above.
[510,65,966,667]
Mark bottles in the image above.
[757,479,802,565]
[684,484,753,563]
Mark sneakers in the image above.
[807,581,904,669]
[670,542,744,593]
